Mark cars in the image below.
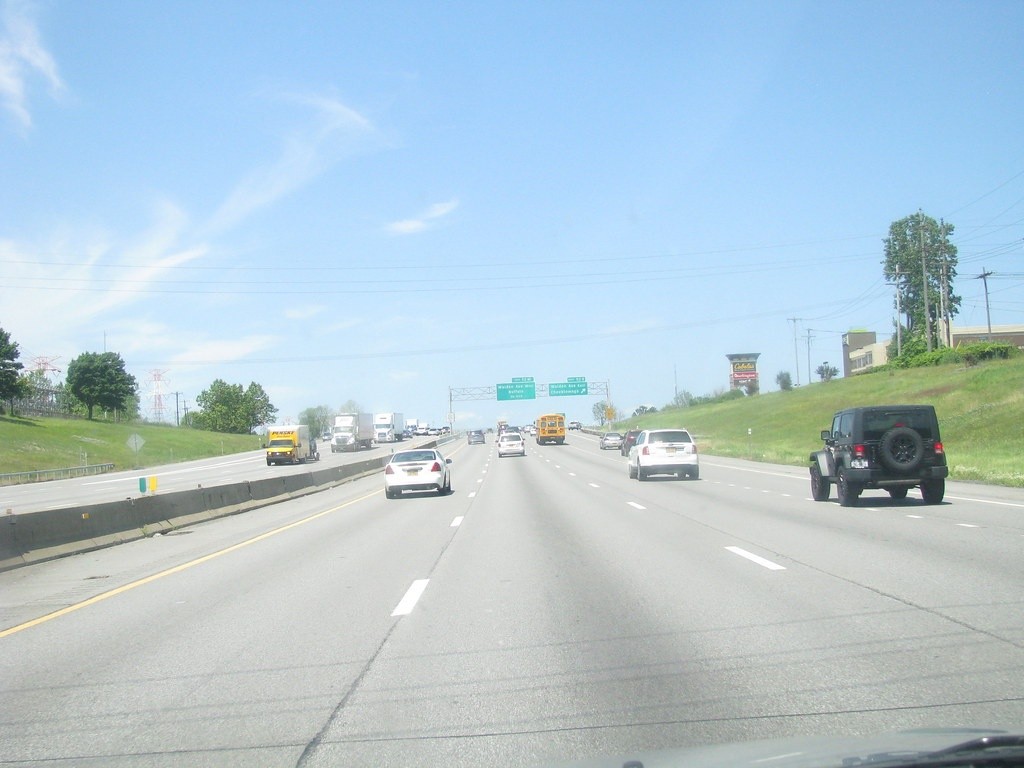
[414,427,450,436]
[310,437,317,458]
[628,429,700,481]
[487,424,536,437]
[384,448,452,499]
[467,430,486,444]
[402,430,413,438]
[495,433,525,458]
[619,430,644,457]
[600,432,623,450]
[322,432,332,442]
[568,421,581,430]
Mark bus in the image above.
[535,414,566,445]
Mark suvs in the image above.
[810,404,949,508]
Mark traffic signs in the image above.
[550,382,588,395]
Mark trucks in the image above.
[262,424,311,466]
[374,412,403,444]
[331,413,374,452]
